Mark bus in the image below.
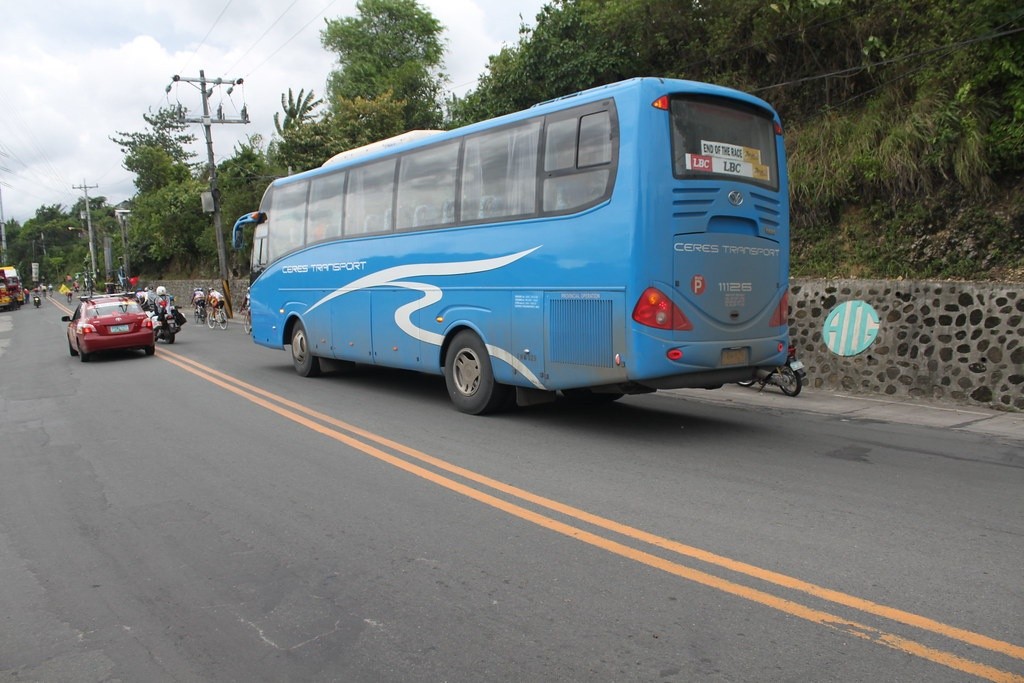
[231,76,792,417]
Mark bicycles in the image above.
[190,301,207,325]
[238,305,252,335]
[205,303,228,330]
[33,286,81,308]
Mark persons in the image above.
[7,273,95,312]
[239,286,252,332]
[197,287,206,312]
[208,287,225,319]
[190,287,205,315]
[133,286,180,333]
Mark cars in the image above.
[60,296,155,362]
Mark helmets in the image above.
[156,286,166,295]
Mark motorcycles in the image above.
[734,338,807,398]
[143,304,181,344]
